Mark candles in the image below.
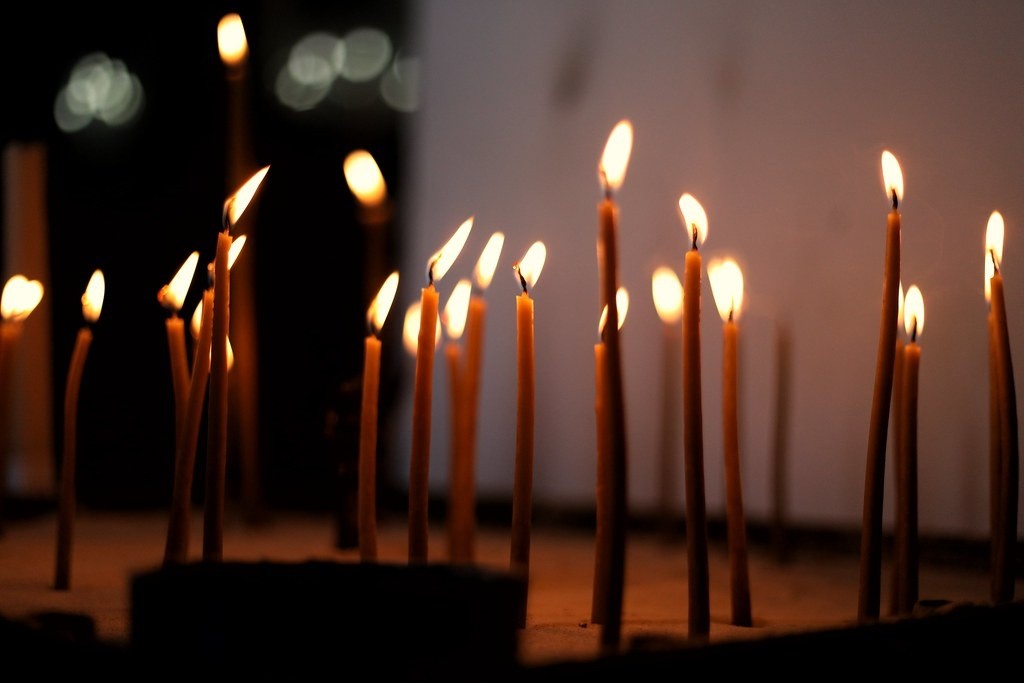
[0,13,1021,658]
